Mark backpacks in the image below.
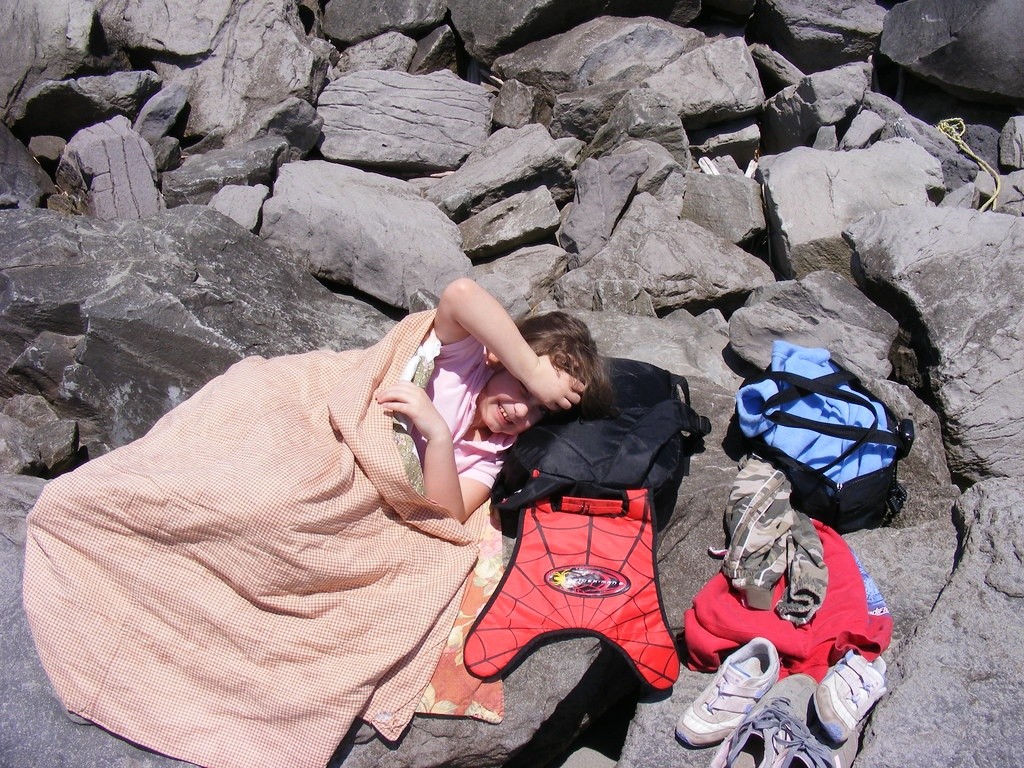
[462,353,712,689]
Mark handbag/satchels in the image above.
[721,341,913,534]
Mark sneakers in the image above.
[813,648,888,742]
[709,672,818,768]
[677,637,779,747]
[772,724,859,768]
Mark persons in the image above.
[374,278,611,527]
